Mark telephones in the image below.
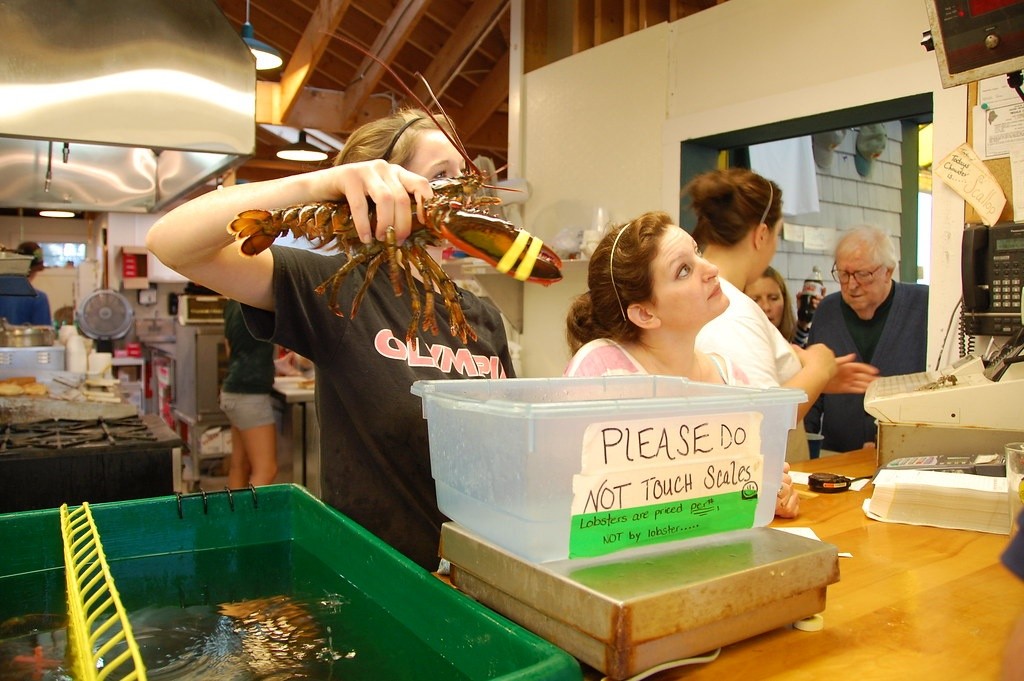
[955,220,1024,336]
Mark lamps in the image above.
[276,131,329,162]
[238,0,285,72]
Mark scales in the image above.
[429,518,842,681]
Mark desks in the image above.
[270,374,315,488]
[427,444,1022,680]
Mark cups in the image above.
[1004,442,1023,544]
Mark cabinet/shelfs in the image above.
[170,324,231,494]
[1,410,183,512]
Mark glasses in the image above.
[831,261,883,284]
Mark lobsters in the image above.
[227,30,564,352]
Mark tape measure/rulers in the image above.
[806,470,853,494]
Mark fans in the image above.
[76,289,134,340]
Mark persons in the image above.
[562,211,799,519]
[679,169,880,427]
[145,103,517,574]
[218,300,276,490]
[802,223,929,459]
[744,267,826,348]
[14,241,50,326]
[1000,511,1023,681]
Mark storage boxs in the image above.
[2,479,582,680]
[410,369,810,565]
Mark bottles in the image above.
[798,266,824,323]
